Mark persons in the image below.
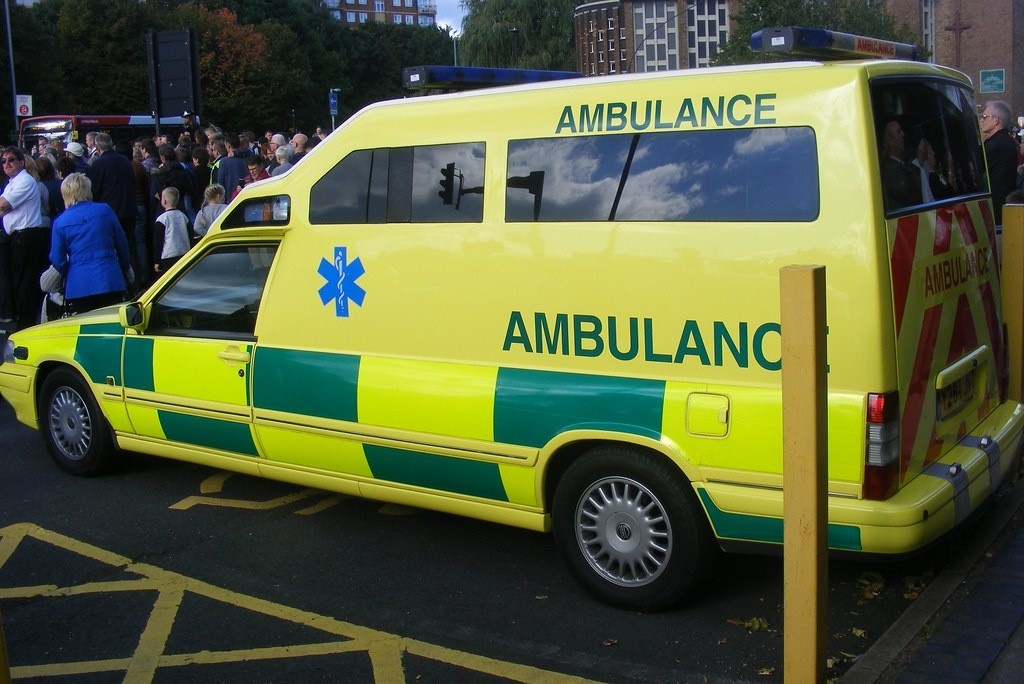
[87,132,138,268]
[193,182,232,238]
[152,187,194,283]
[50,172,130,314]
[149,144,196,228]
[0,146,42,335]
[0,109,332,342]
[880,119,981,212]
[967,100,1024,226]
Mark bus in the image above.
[19,113,201,158]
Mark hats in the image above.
[181,110,195,118]
[63,142,84,157]
[1012,125,1021,133]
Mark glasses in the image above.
[0,157,19,164]
[982,114,994,118]
[268,140,276,144]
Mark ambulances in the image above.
[0,27,1024,613]
